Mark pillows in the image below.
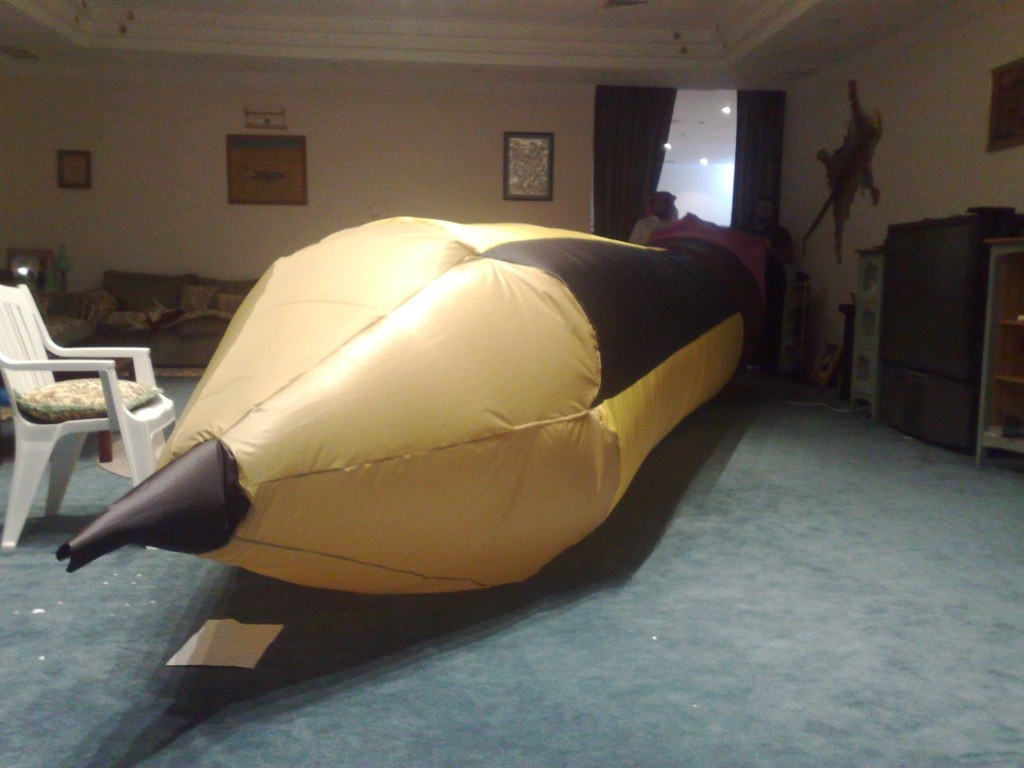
[100,269,259,332]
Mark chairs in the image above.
[1,283,177,550]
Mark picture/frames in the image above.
[501,132,553,200]
[988,56,1024,152]
[7,248,54,295]
[56,148,91,188]
[226,134,308,205]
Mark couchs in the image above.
[32,290,234,368]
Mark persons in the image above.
[736,195,794,375]
[628,192,678,246]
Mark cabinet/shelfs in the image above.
[849,250,888,424]
[764,279,810,380]
[973,232,1024,469]
